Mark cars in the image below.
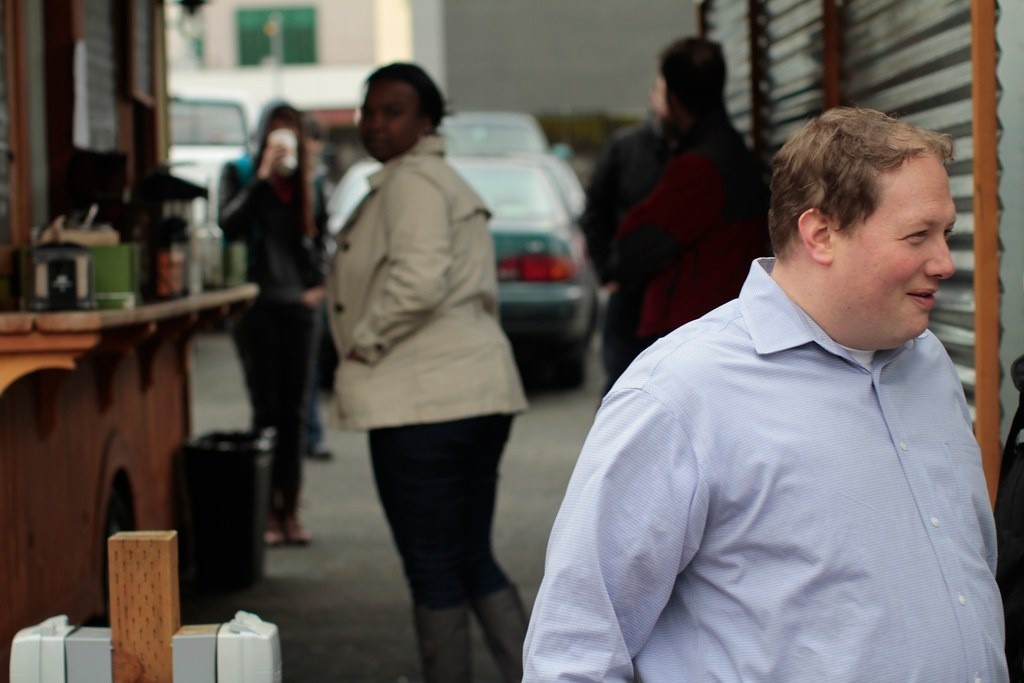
[434,111,577,174]
[324,158,602,385]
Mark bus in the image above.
[166,97,259,290]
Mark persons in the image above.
[327,60,528,683]
[578,36,771,404]
[523,107,1009,683]
[218,100,357,547]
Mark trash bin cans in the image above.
[179,428,276,598]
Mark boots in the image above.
[415,605,472,683]
[471,583,529,683]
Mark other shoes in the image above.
[257,511,284,545]
[300,448,334,461]
[281,513,308,542]
